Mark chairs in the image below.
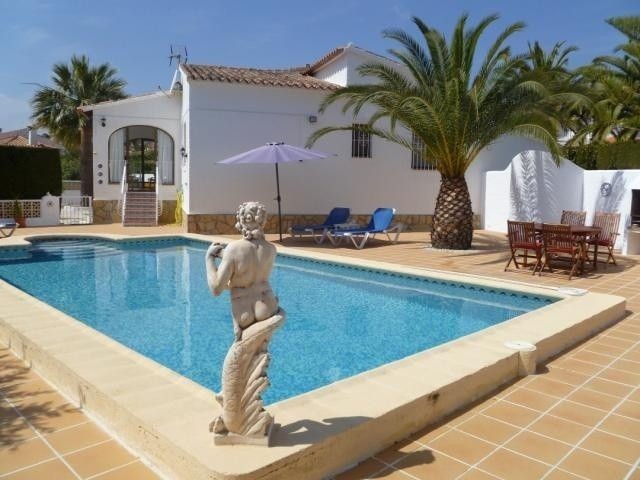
[0,218,20,237]
[505,210,621,281]
[326,208,403,250]
[288,208,350,244]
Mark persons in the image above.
[204,200,280,328]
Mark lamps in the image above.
[310,116,316,122]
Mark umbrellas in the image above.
[213,140,338,241]
